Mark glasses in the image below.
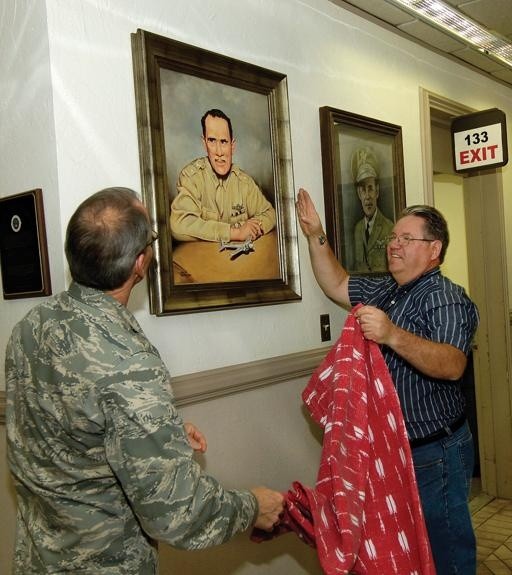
[384,233,435,246]
[135,229,163,261]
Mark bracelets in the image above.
[316,232,328,248]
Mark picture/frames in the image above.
[0,189,52,299]
[131,28,302,317]
[320,106,407,278]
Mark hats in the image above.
[355,163,378,183]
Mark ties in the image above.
[364,223,371,246]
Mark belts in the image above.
[407,410,470,452]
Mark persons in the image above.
[0,186,284,574]
[351,146,395,270]
[170,108,277,242]
[293,186,480,575]
